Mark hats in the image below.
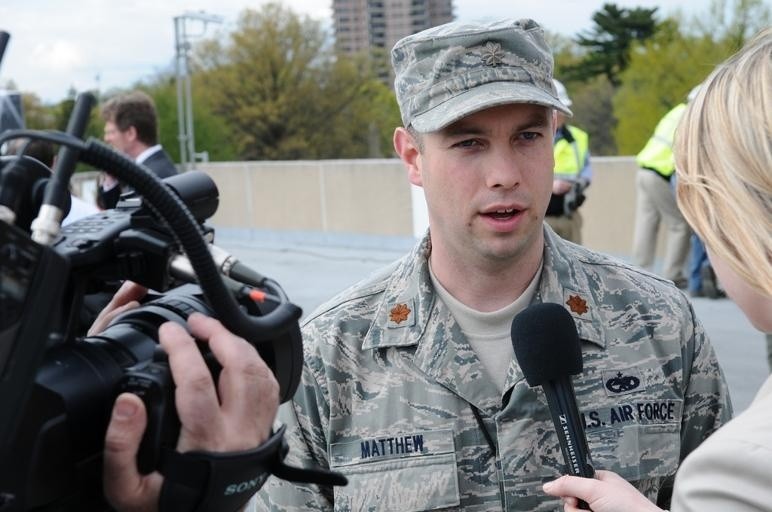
[390,17,572,133]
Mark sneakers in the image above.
[674,267,726,299]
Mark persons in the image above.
[94,88,177,211]
[630,81,706,289]
[253,13,733,511]
[540,27,771,512]
[85,272,284,512]
[538,79,594,252]
[687,233,727,300]
[11,140,100,228]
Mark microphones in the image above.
[511,302,596,512]
